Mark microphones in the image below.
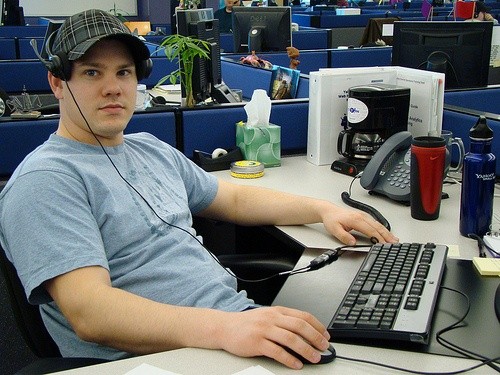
[30,38,56,72]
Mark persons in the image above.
[474,1,498,24]
[0,9,398,370]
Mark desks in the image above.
[45,153,500,375]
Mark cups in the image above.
[136,84,149,111]
[410,135,452,222]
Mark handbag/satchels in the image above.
[271,79,288,99]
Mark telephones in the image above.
[360,130,449,207]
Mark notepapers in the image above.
[473,257,500,275]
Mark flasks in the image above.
[460,115,496,240]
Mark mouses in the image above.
[278,335,336,364]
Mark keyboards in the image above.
[325,241,449,353]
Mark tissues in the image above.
[235,88,282,168]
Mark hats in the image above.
[51,9,150,63]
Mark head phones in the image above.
[44,29,156,80]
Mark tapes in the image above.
[212,148,228,158]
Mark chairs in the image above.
[124,21,151,35]
[0,179,296,356]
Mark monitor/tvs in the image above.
[393,21,493,90]
[0,0,25,26]
[231,6,292,53]
[176,8,239,104]
